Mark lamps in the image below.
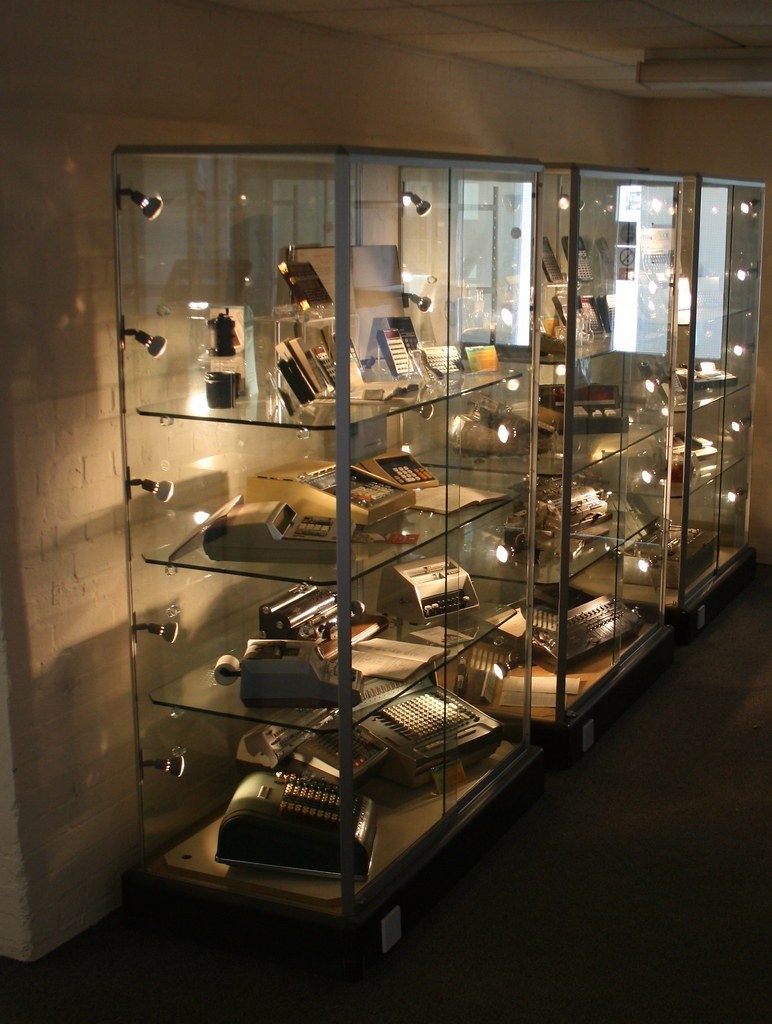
[636,45,772,92]
